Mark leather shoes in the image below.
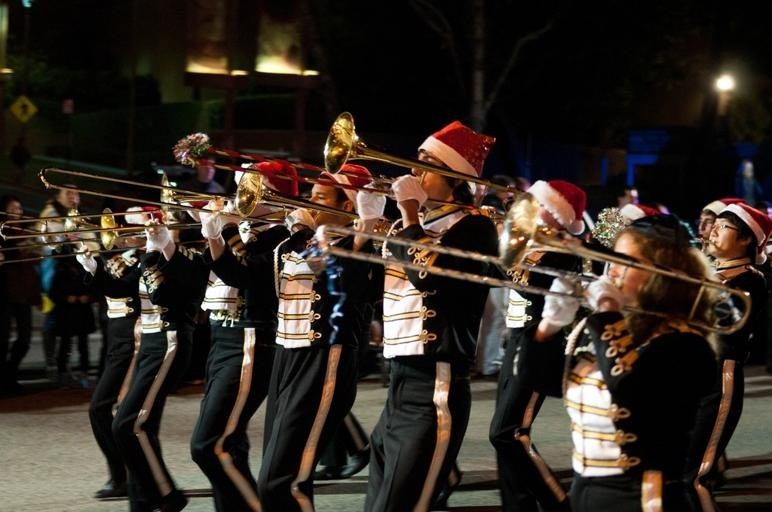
[313,444,370,480]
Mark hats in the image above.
[417,120,495,178]
[528,179,587,229]
[620,203,659,221]
[703,198,772,265]
[247,157,375,211]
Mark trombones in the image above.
[314,209,750,332]
[0,111,534,264]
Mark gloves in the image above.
[76,244,98,273]
[356,175,426,219]
[145,201,316,251]
[541,278,624,328]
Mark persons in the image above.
[2,119,772,512]
